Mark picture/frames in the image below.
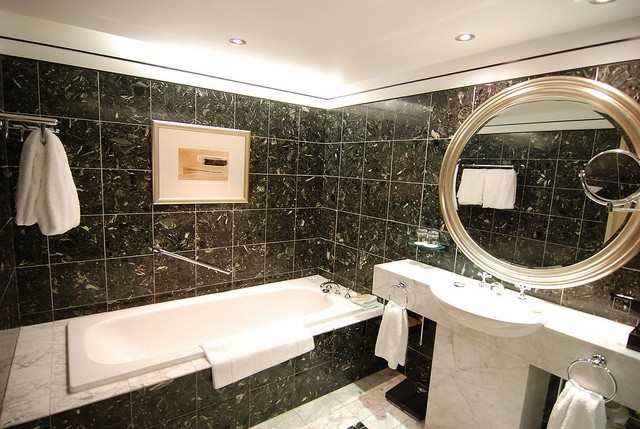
[150,118,252,207]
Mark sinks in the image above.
[437,281,539,323]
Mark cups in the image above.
[416,229,427,242]
[427,230,440,246]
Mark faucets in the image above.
[489,282,505,294]
[319,280,340,291]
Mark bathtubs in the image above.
[66,281,364,394]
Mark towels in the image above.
[374,303,408,368]
[14,125,83,236]
[547,376,607,429]
[199,321,314,390]
[456,168,485,206]
[483,170,517,210]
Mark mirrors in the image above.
[438,74,640,291]
[579,149,640,208]
